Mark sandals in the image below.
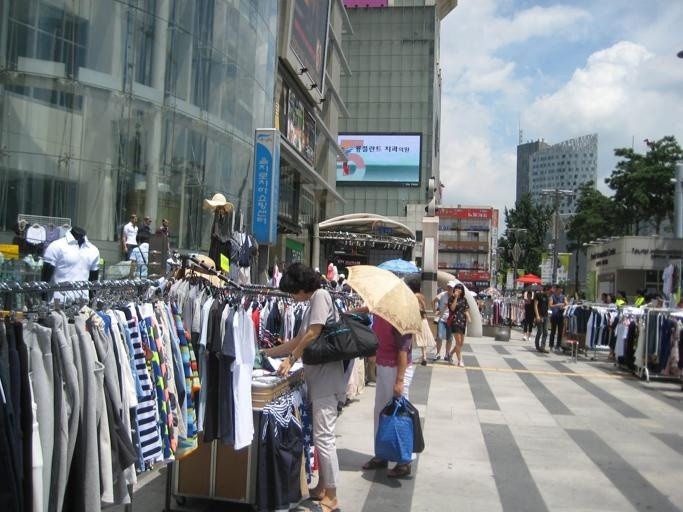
[387,462,411,477]
[363,457,388,469]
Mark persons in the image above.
[602,288,646,307]
[350,306,415,478]
[41,228,101,306]
[264,265,340,511]
[122,214,178,279]
[409,279,494,367]
[521,284,567,354]
[320,262,352,300]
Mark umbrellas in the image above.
[377,258,420,272]
[516,273,544,284]
[347,262,423,335]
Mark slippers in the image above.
[303,501,340,511]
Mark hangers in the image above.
[0,279,171,330]
[181,252,308,312]
[18,218,71,231]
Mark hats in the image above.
[203,194,234,212]
[447,280,455,287]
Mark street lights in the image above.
[506,225,528,290]
[540,186,574,285]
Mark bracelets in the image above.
[288,352,297,365]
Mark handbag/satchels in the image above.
[375,416,413,462]
[302,312,379,366]
[534,316,544,324]
[447,315,454,327]
[433,314,441,324]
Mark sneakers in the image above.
[538,347,562,353]
[433,354,464,367]
[421,360,427,365]
[523,335,531,341]
